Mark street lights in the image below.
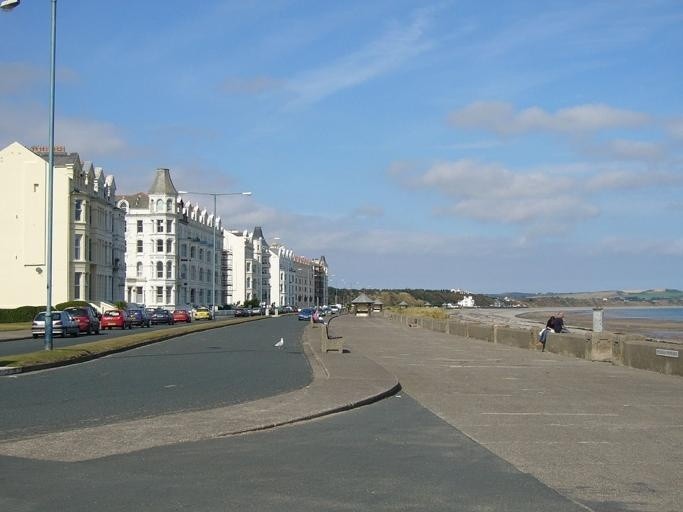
[175,189,254,320]
[0,0,61,351]
[237,236,282,317]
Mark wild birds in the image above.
[273,337,284,350]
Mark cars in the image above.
[193,306,212,322]
[150,308,175,326]
[62,304,101,337]
[233,303,344,322]
[31,309,79,339]
[172,308,192,324]
[100,309,132,330]
[125,307,152,329]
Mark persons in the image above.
[553,313,575,335]
[546,313,559,330]
